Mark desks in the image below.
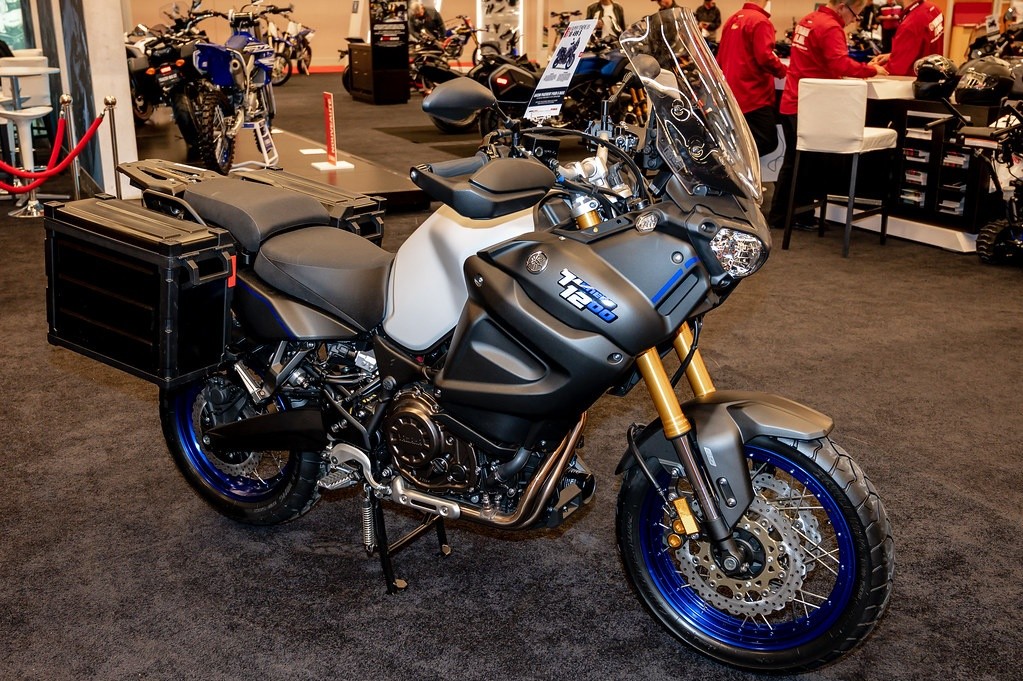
[0,64,61,107]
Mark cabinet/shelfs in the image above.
[348,41,413,106]
[769,71,1007,252]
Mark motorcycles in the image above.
[41,6,898,674]
[124,1,1021,266]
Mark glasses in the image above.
[845,4,862,22]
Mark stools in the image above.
[1,94,39,194]
[0,101,59,219]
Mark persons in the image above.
[867,0,945,78]
[1003,13,1017,31]
[763,0,890,233]
[586,0,626,41]
[651,0,680,12]
[715,0,788,159]
[694,0,722,43]
[407,0,446,98]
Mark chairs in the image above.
[781,79,898,260]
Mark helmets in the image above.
[956,55,1015,103]
[912,54,955,98]
